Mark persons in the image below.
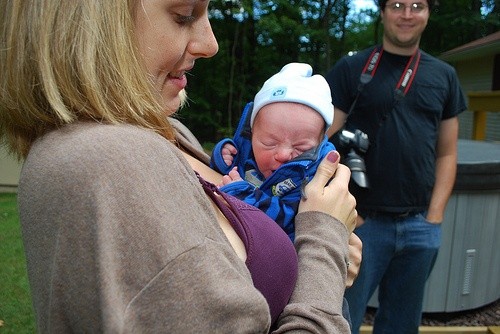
[0,0,363,334]
[213,62,338,242]
[324,0,468,334]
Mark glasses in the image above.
[385,3,428,12]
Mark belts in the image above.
[359,208,423,223]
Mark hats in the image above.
[250,62,335,131]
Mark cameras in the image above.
[332,128,371,190]
